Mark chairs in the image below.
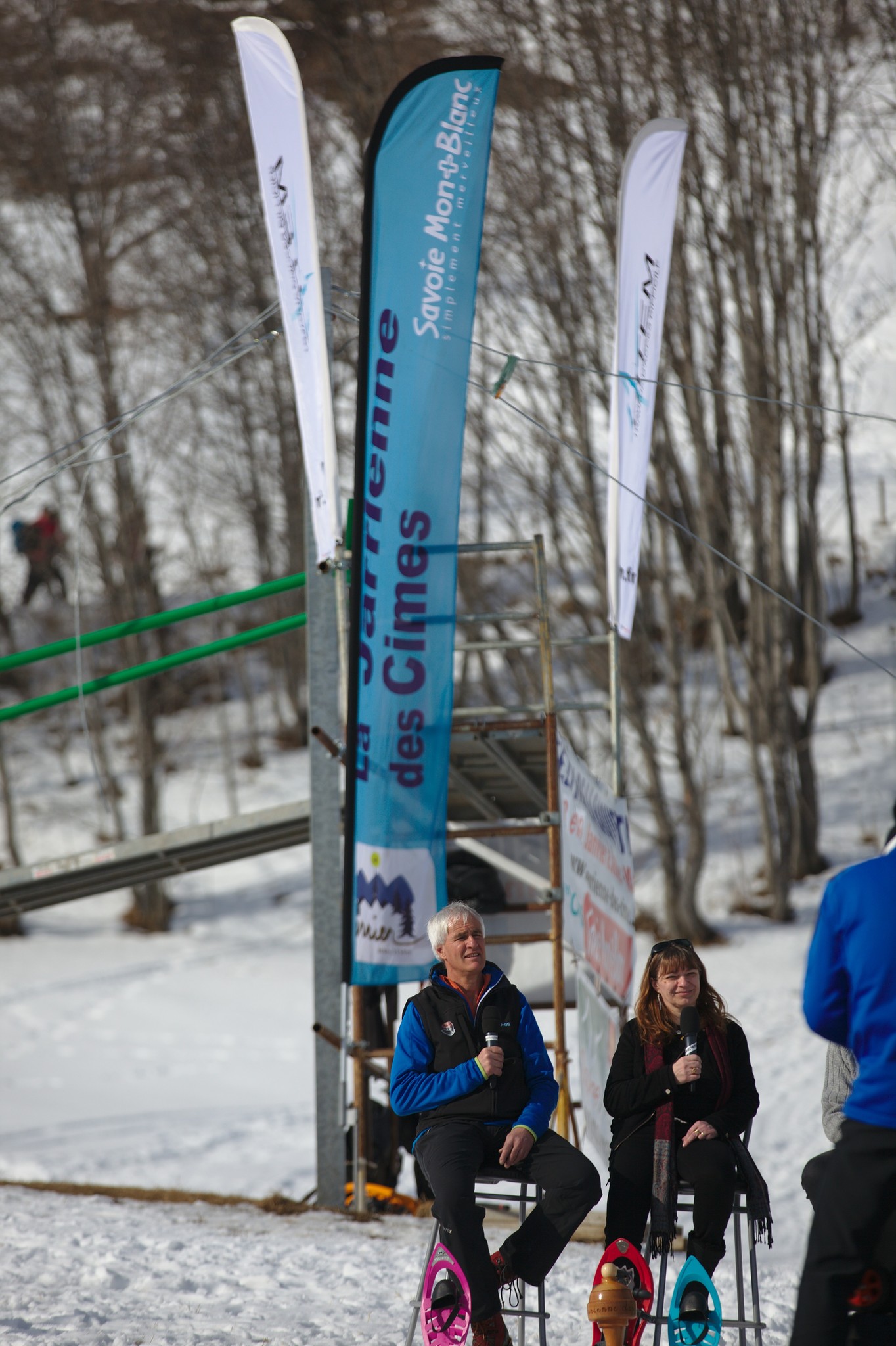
[642,1112,769,1346]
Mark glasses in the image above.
[650,937,695,957]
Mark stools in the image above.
[404,1168,555,1346]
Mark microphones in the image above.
[482,1005,502,1089]
[680,1006,701,1092]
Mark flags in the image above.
[340,51,505,988]
[229,14,346,580]
[609,117,695,642]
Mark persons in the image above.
[818,1036,861,1148]
[787,802,896,1346]
[392,900,604,1346]
[602,935,762,1321]
[12,506,66,615]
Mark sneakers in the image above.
[490,1251,517,1289]
[470,1313,511,1345]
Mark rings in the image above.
[701,1132,706,1137]
[694,1129,701,1135]
[690,1067,697,1073]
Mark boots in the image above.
[680,1233,725,1320]
